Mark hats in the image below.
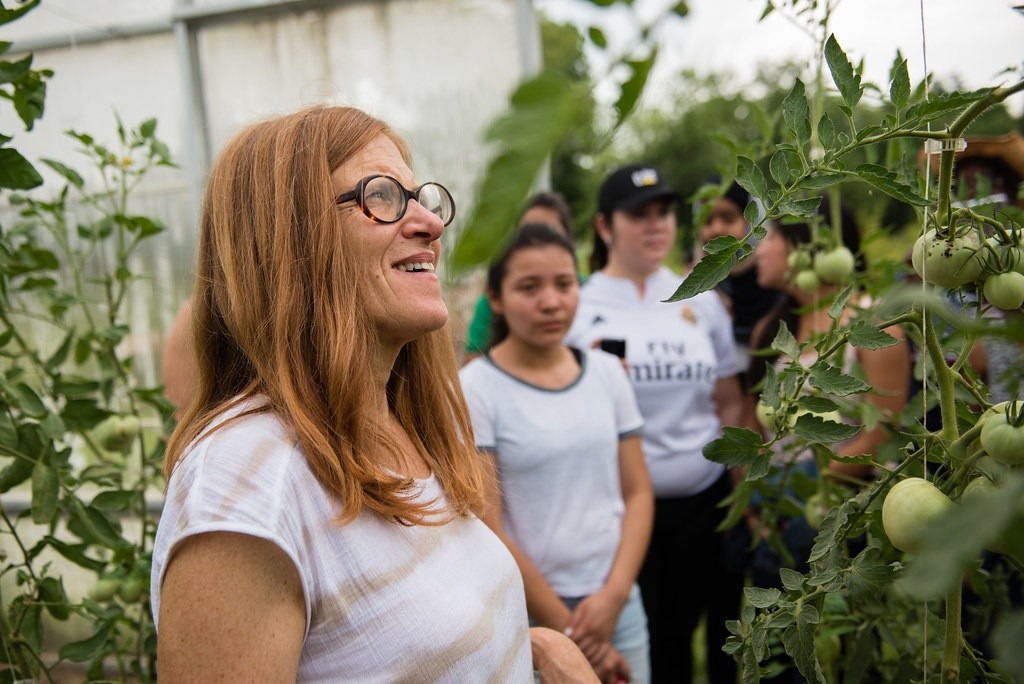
[597,165,683,209]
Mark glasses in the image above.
[333,174,455,229]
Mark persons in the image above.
[562,162,751,684]
[696,132,1024,684]
[151,106,535,684]
[451,223,655,684]
[463,192,589,367]
